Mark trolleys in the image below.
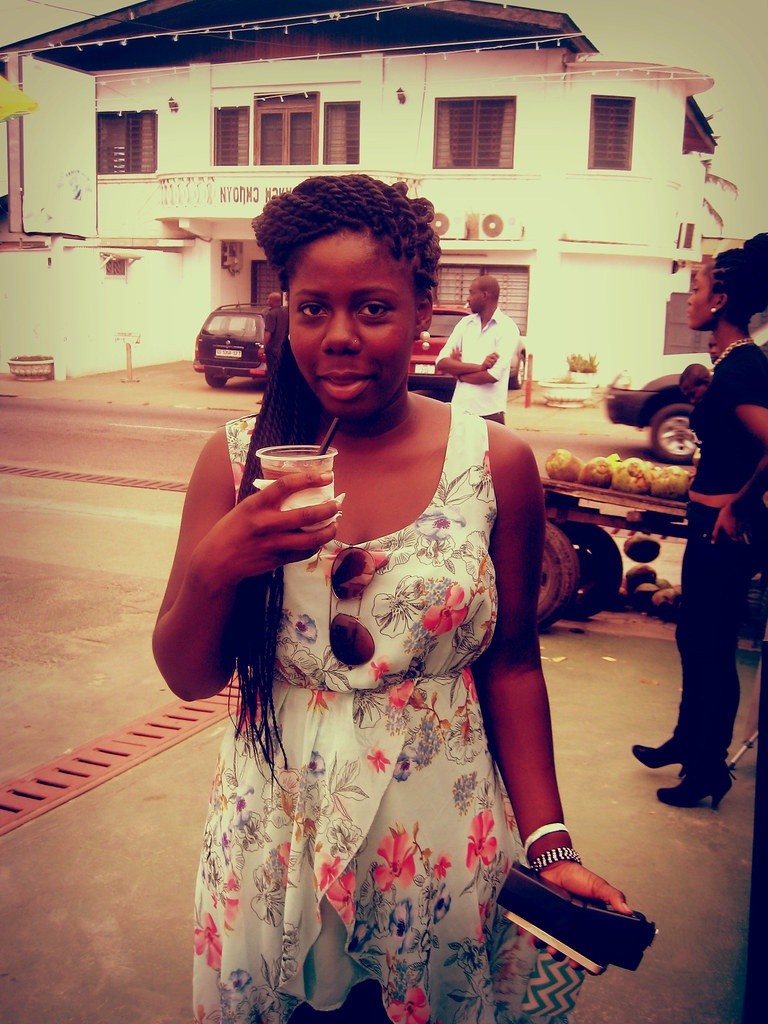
[536,473,690,629]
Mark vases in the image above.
[8,354,56,383]
[535,378,596,410]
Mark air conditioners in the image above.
[428,210,467,240]
[477,212,522,240]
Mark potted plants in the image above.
[564,353,601,387]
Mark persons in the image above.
[256,293,289,403]
[633,233,768,809]
[150,175,637,1024]
[708,333,723,364]
[679,363,712,405]
[435,274,520,423]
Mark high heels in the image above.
[634,733,733,811]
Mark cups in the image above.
[255,442,338,531]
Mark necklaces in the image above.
[712,338,754,364]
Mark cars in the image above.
[404,304,526,390]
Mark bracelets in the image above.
[523,822,569,866]
[531,847,583,871]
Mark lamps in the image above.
[168,97,180,112]
[396,87,406,104]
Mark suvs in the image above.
[604,324,768,463]
[191,302,270,389]
